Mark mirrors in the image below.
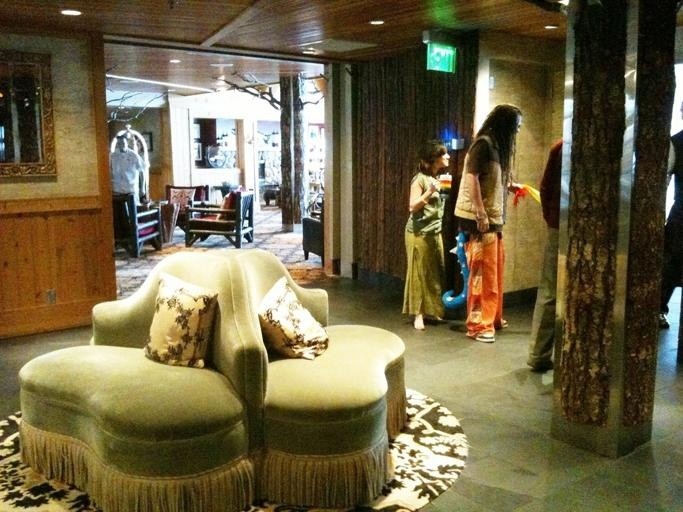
[0,49,59,179]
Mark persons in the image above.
[454,104,526,343]
[401,139,451,331]
[109,134,146,206]
[527,138,563,371]
[665,103,683,315]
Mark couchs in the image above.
[16,247,408,512]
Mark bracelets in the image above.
[420,197,427,206]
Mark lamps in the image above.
[421,30,430,44]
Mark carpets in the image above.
[0,387,469,512]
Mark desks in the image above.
[135,201,181,243]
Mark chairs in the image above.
[175,185,210,241]
[302,197,324,267]
[113,193,163,259]
[184,188,254,248]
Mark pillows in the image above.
[165,184,205,215]
[216,184,244,222]
[258,275,329,360]
[144,270,220,371]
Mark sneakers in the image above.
[659,313,669,329]
[476,331,495,343]
[528,360,553,371]
[501,318,509,327]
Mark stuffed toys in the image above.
[442,226,471,310]
